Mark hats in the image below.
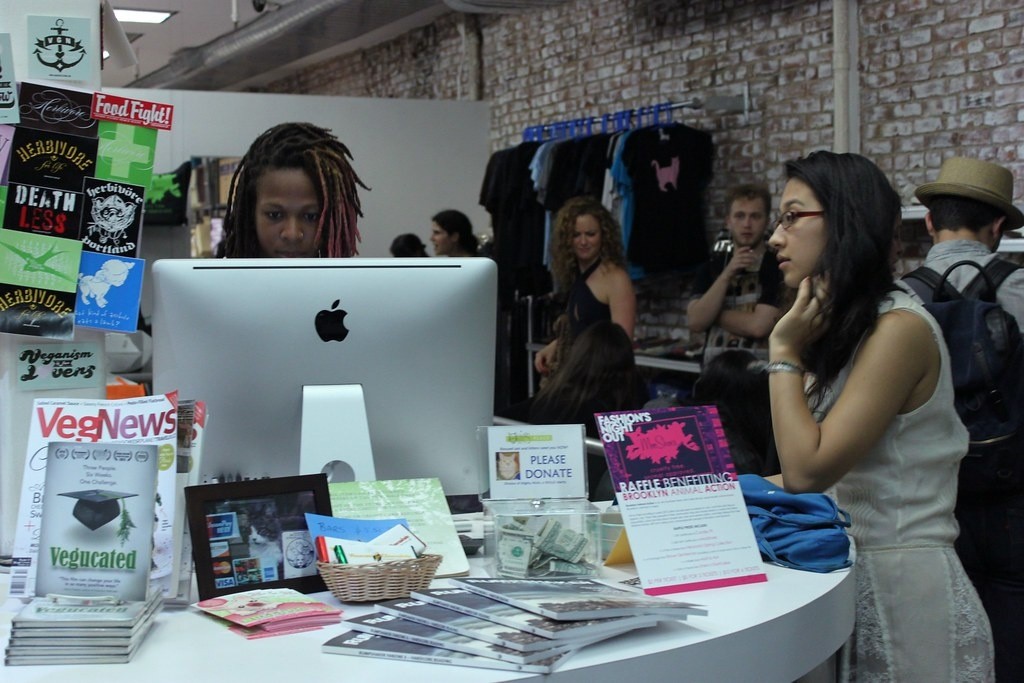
[914,157,1024,230]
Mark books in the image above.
[4,585,165,666]
[6,390,208,609]
[320,575,710,674]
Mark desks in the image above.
[527,339,702,377]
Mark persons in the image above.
[222,122,372,259]
[900,156,1024,683]
[389,233,430,257]
[685,184,786,374]
[691,350,781,477]
[534,195,637,376]
[767,150,996,683]
[526,319,649,502]
[429,210,481,257]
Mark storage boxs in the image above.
[475,424,601,579]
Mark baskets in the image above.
[315,553,443,601]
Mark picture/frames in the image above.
[184,473,333,603]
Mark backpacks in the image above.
[900,258,1024,495]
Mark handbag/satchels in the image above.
[738,474,853,574]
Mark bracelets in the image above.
[765,360,805,377]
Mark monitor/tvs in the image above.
[154,258,498,522]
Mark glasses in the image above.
[774,210,824,229]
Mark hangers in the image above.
[522,100,673,144]
